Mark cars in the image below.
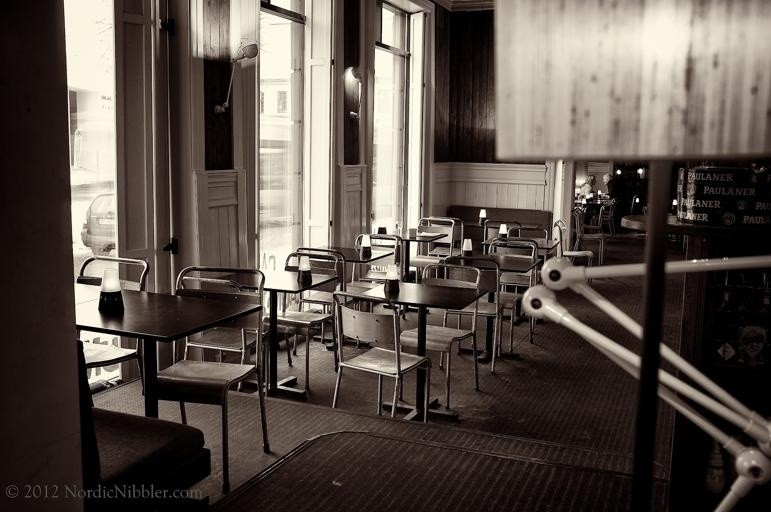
[81,194,115,256]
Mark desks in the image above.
[455,255,542,326]
[481,236,560,263]
[364,283,490,420]
[309,247,394,349]
[213,273,338,400]
[370,234,448,315]
[619,214,699,265]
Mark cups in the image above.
[395,220,403,238]
[408,228,418,238]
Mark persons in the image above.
[603,173,630,211]
[579,175,598,198]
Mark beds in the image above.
[76,283,262,418]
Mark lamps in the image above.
[217,44,257,112]
[463,239,472,251]
[479,209,486,228]
[499,224,508,237]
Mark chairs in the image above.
[507,226,549,294]
[488,241,535,342]
[332,292,429,421]
[354,233,402,335]
[157,268,269,491]
[398,265,478,410]
[426,217,463,278]
[584,206,604,233]
[416,218,455,283]
[554,220,594,285]
[438,254,504,374]
[590,198,616,237]
[77,340,212,512]
[352,235,403,348]
[571,208,606,264]
[77,256,153,396]
[283,253,338,372]
[292,249,346,354]
[492,238,538,334]
[483,220,520,255]
[184,327,258,389]
